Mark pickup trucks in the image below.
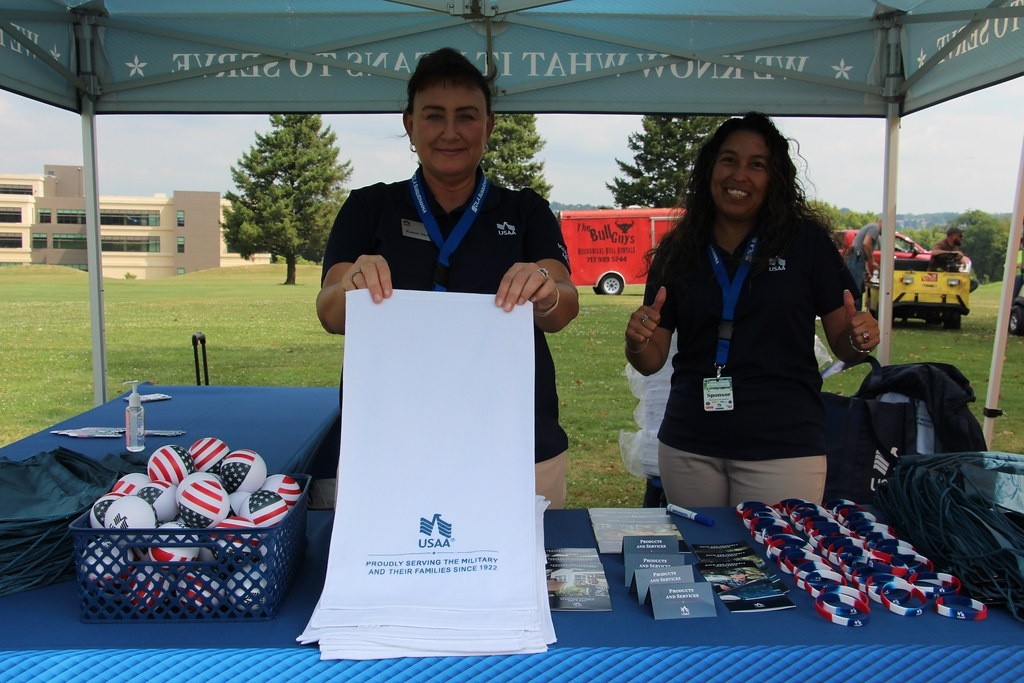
[865,268,970,325]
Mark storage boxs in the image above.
[66,474,311,627]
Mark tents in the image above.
[0,1,1023,451]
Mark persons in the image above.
[845,218,881,292]
[625,111,880,506]
[316,47,579,509]
[1012,237,1024,301]
[929,227,978,292]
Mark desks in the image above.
[0,386,1024,683]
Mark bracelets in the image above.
[534,289,559,317]
[737,496,987,627]
[848,332,872,354]
[627,338,649,353]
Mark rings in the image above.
[641,316,647,322]
[538,268,548,278]
[351,271,361,278]
[863,331,870,341]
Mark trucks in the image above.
[559,206,972,296]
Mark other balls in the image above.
[83,438,302,613]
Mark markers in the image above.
[666,503,715,527]
